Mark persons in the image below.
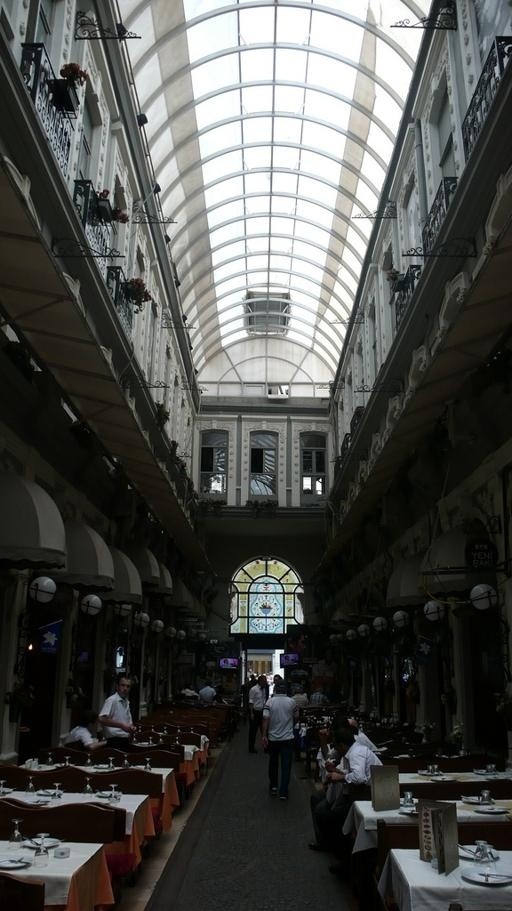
[223,673,235,689]
[181,683,200,706]
[308,730,384,851]
[98,676,141,750]
[64,710,107,750]
[319,715,380,764]
[247,674,344,802]
[199,680,217,706]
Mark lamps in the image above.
[327,583,512,685]
[13,575,206,673]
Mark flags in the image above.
[38,620,62,656]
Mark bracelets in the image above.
[320,744,328,752]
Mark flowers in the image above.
[386,268,399,282]
[135,277,151,302]
[61,60,88,85]
[99,188,129,223]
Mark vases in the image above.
[391,273,405,291]
[59,79,79,110]
[128,283,138,301]
[98,198,114,222]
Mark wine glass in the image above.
[109,784,120,804]
[82,776,93,801]
[477,845,498,877]
[33,832,50,869]
[50,783,63,806]
[0,779,7,798]
[8,818,24,849]
[18,725,196,774]
[24,776,36,800]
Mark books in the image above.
[418,799,459,875]
[370,765,400,811]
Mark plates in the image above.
[95,789,124,798]
[461,796,495,804]
[23,798,50,806]
[418,770,444,775]
[459,843,500,861]
[0,853,33,869]
[461,865,512,885]
[485,774,511,780]
[36,789,63,796]
[22,837,61,848]
[474,769,498,775]
[398,797,420,815]
[0,787,14,793]
[431,776,457,781]
[473,806,510,813]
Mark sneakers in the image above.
[249,749,257,753]
[270,787,288,800]
[307,838,328,851]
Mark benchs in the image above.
[0,696,234,911]
[295,701,512,911]
